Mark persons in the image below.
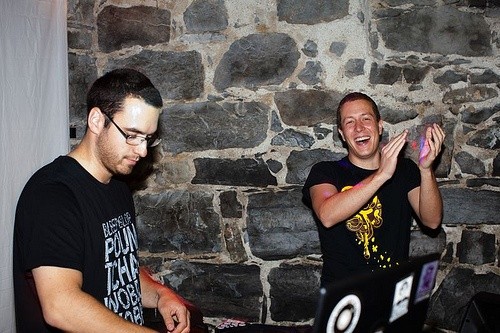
[301,92,449,333]
[12,66,196,333]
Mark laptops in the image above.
[311,251,444,333]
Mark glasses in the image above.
[102,110,163,148]
